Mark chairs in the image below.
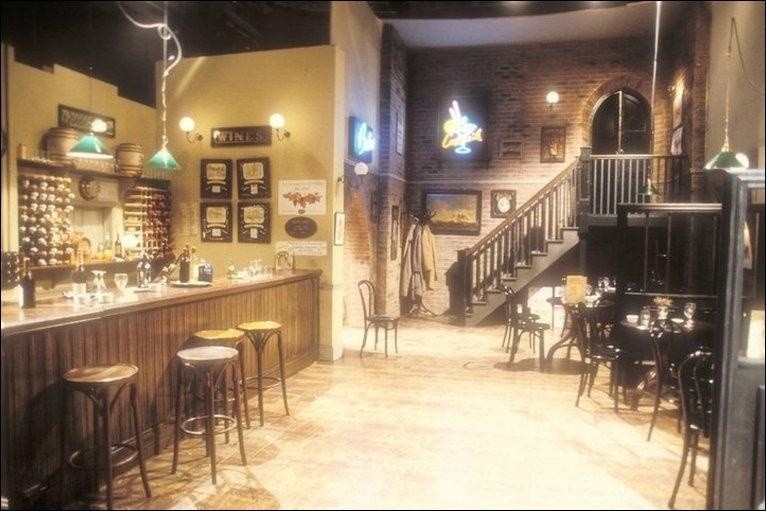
[501,282,550,371]
[356,279,400,361]
[667,346,719,509]
[646,319,687,441]
[569,297,629,413]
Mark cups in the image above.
[230,260,273,279]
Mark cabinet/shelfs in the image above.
[15,159,72,267]
[121,176,175,256]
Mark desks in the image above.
[546,295,617,368]
[612,316,716,411]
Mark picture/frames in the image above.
[199,202,233,244]
[57,102,116,138]
[540,126,566,164]
[237,201,271,244]
[333,210,346,247]
[209,124,272,148]
[200,158,232,200]
[236,157,272,199]
[498,138,525,160]
[422,190,482,237]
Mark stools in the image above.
[234,318,290,429]
[171,348,247,486]
[192,328,247,446]
[58,362,151,510]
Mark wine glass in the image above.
[90,270,110,304]
[113,272,129,301]
[589,276,616,300]
[642,303,699,333]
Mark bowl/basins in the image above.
[625,315,639,323]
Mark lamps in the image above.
[631,1,664,197]
[546,90,560,112]
[146,1,182,171]
[66,0,114,159]
[178,116,203,145]
[268,112,292,142]
[336,160,369,189]
[703,15,748,172]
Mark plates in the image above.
[172,279,211,287]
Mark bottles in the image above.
[18,175,75,267]
[72,249,86,303]
[127,183,172,259]
[137,251,151,290]
[180,247,200,284]
[20,257,37,312]
[97,233,123,262]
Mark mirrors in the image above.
[490,189,516,219]
